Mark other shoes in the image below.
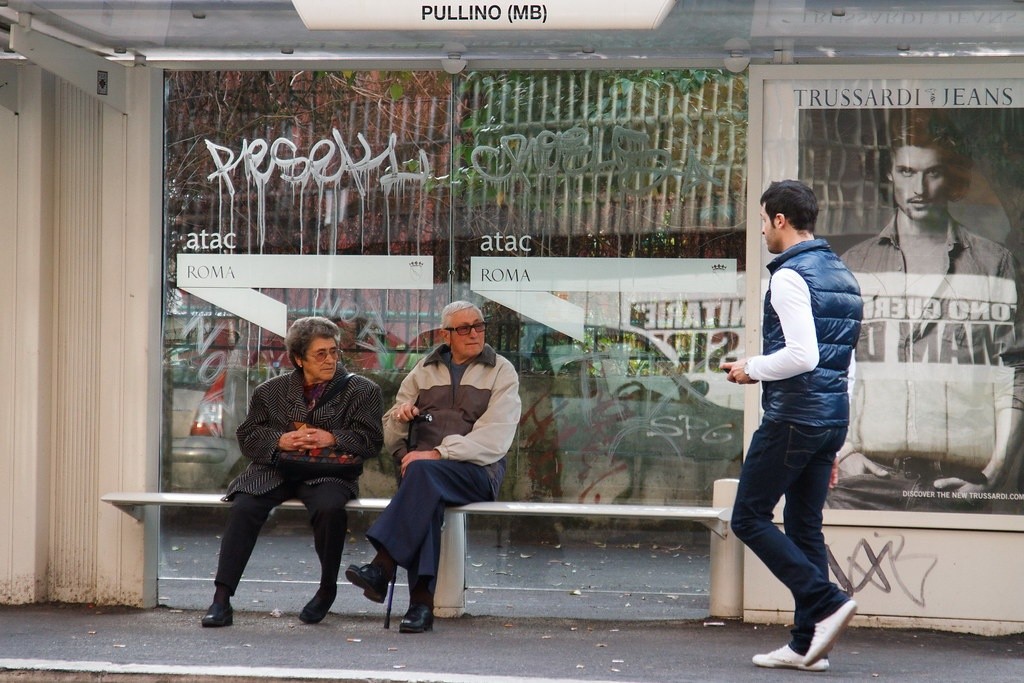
[297,588,336,624]
[201,602,234,626]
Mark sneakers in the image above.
[750,643,830,671]
[801,597,858,667]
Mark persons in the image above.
[345,300,523,633]
[719,179,864,670]
[835,127,1024,510]
[203,317,384,634]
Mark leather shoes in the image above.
[398,601,434,632]
[345,563,391,603]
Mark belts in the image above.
[868,454,988,485]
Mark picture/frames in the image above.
[744,58,1024,532]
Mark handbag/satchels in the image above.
[279,421,363,481]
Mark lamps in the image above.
[439,56,467,75]
[442,42,466,56]
[722,39,750,53]
[724,53,751,74]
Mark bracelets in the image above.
[744,363,748,374]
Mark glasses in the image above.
[307,347,344,362]
[444,321,487,335]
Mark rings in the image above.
[307,435,309,437]
[397,414,400,419]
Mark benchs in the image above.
[102,478,744,619]
